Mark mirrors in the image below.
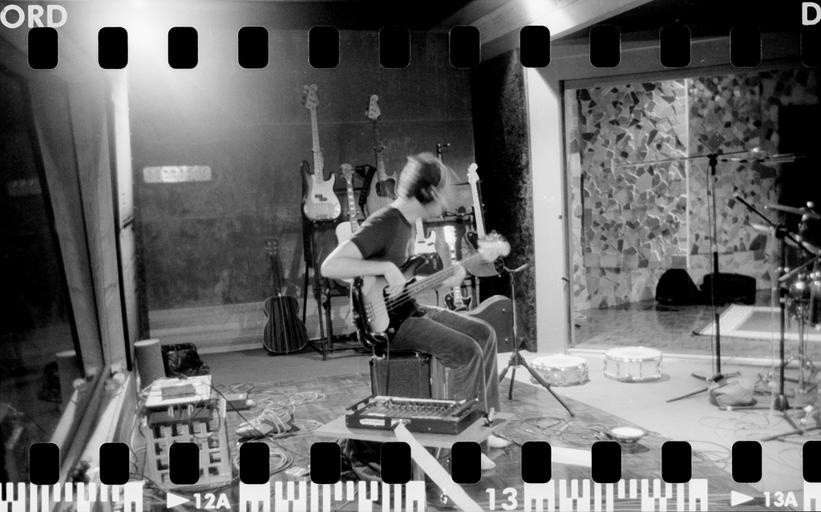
[0,21,104,494]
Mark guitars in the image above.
[412,217,471,312]
[335,163,361,288]
[461,163,505,277]
[350,233,511,351]
[365,94,399,219]
[302,83,341,222]
[263,239,309,354]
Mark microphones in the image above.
[766,154,798,164]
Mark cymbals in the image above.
[765,204,820,219]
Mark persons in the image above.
[319,146,513,475]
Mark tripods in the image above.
[497,285,575,419]
[665,195,821,442]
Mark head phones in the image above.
[415,159,445,203]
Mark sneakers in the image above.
[481,451,496,470]
[486,433,515,449]
